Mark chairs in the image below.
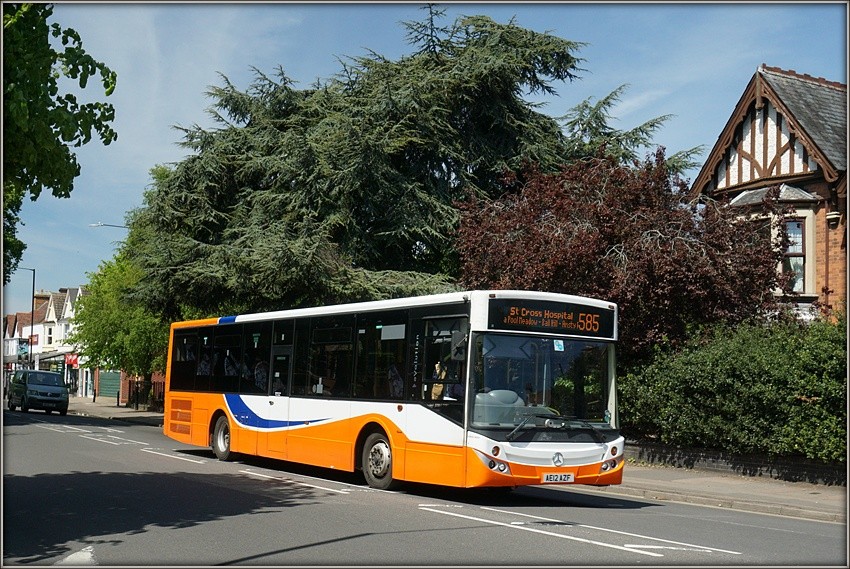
[30,375,57,385]
[185,340,450,400]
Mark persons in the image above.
[452,362,491,424]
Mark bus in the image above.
[162,287,625,494]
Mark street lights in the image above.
[11,266,36,367]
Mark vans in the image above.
[7,368,72,417]
